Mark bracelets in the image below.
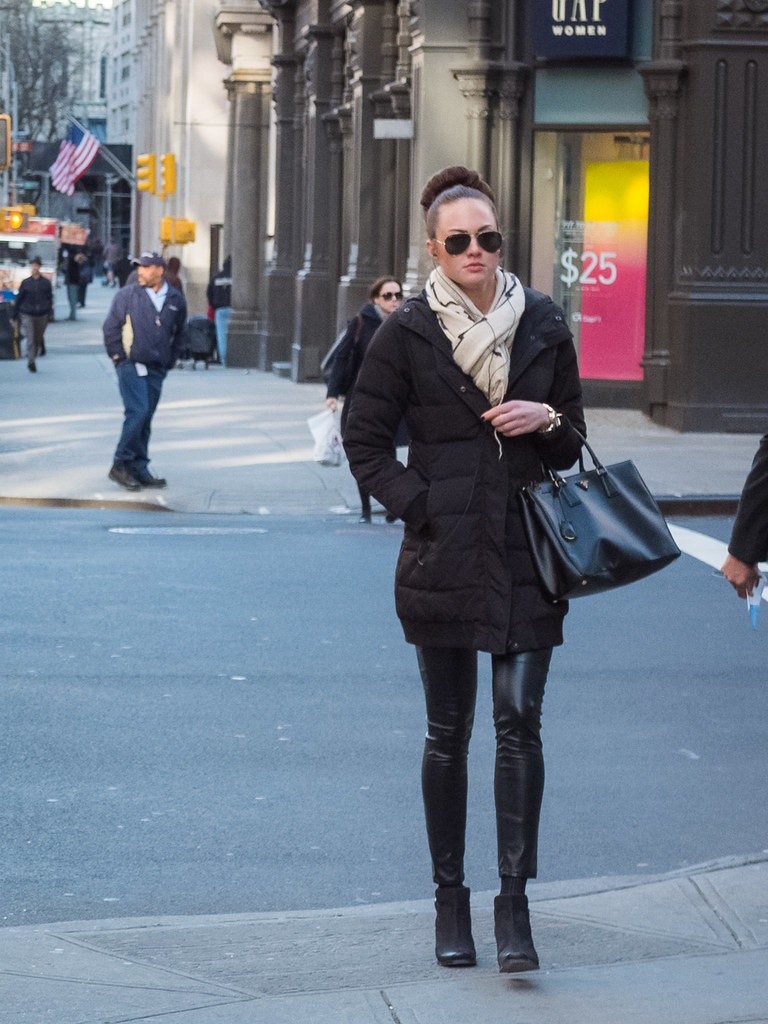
[543,403,562,431]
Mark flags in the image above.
[48,122,100,196]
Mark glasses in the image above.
[430,231,503,255]
[377,292,404,302]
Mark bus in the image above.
[0,203,63,303]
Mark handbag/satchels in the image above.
[307,409,347,467]
[321,311,364,398]
[80,265,92,284]
[520,427,680,605]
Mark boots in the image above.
[493,893,541,973]
[433,887,476,965]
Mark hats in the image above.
[130,251,165,267]
[27,255,42,265]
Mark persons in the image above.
[102,252,188,490]
[328,277,403,524]
[207,255,232,364]
[722,431,768,599]
[65,250,93,320]
[14,256,53,371]
[104,252,132,288]
[342,166,587,974]
[165,257,182,290]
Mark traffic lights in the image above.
[0,115,10,169]
[136,153,156,194]
[161,154,176,194]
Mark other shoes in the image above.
[108,464,141,490]
[28,362,36,372]
[359,515,371,523]
[134,466,166,489]
[38,346,46,356]
[385,512,395,520]
